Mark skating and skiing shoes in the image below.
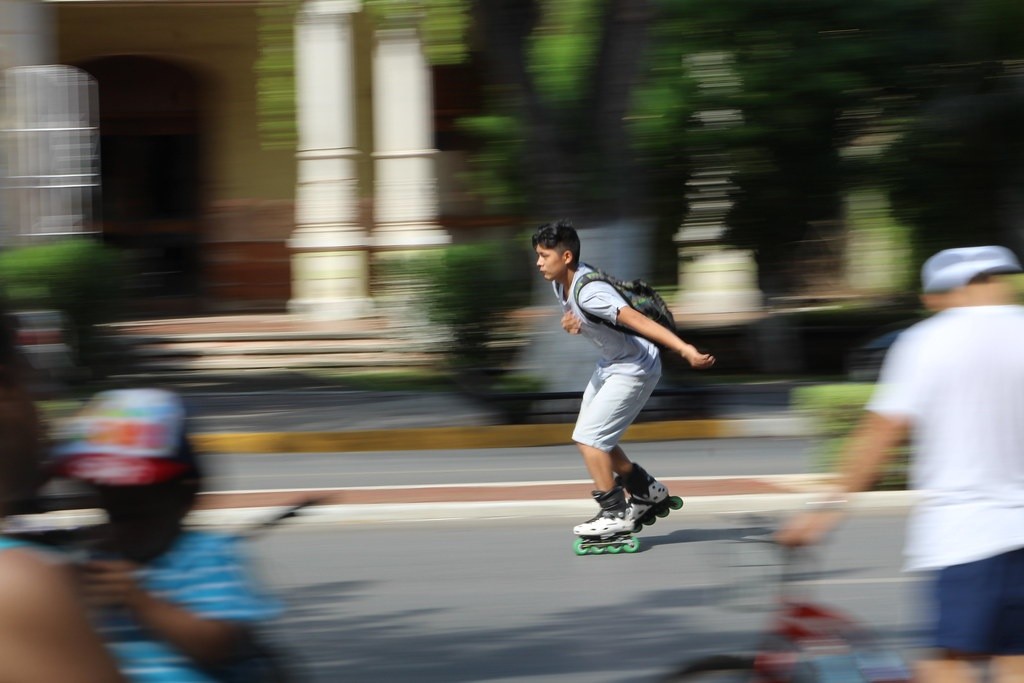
[616,462,683,533]
[573,485,640,555]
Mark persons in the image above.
[52,386,284,683]
[775,246,1024,683]
[532,220,716,555]
[0,311,123,683]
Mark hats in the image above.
[920,245,1024,293]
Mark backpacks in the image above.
[555,259,675,347]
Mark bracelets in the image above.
[802,490,847,511]
